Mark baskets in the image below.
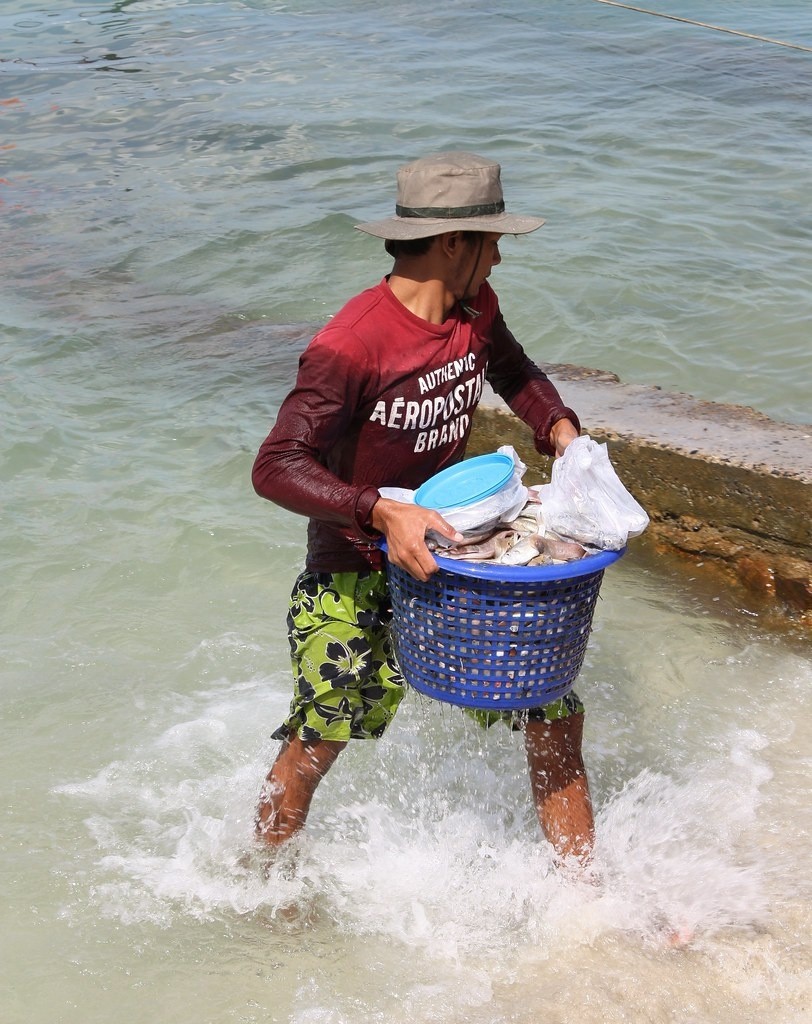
[380,545,627,712]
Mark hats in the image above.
[352,152,545,239]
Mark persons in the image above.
[246,147,615,886]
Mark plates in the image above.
[412,453,515,511]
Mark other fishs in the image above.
[386,489,602,698]
